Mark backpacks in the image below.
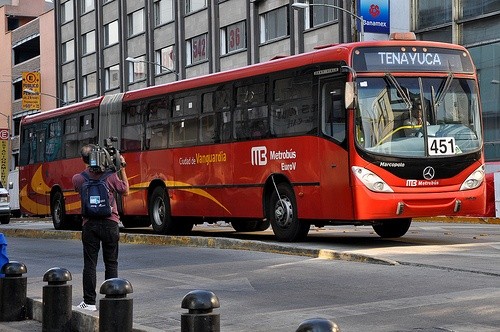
[79,170,112,219]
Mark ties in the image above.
[418,119,421,125]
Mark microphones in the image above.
[109,136,118,141]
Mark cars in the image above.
[0,180,13,225]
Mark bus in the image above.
[18,31,487,244]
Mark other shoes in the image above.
[76,301,97,311]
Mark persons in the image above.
[403,99,430,139]
[70,143,132,313]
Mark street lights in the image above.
[292,2,364,42]
[125,57,180,80]
[23,89,68,105]
